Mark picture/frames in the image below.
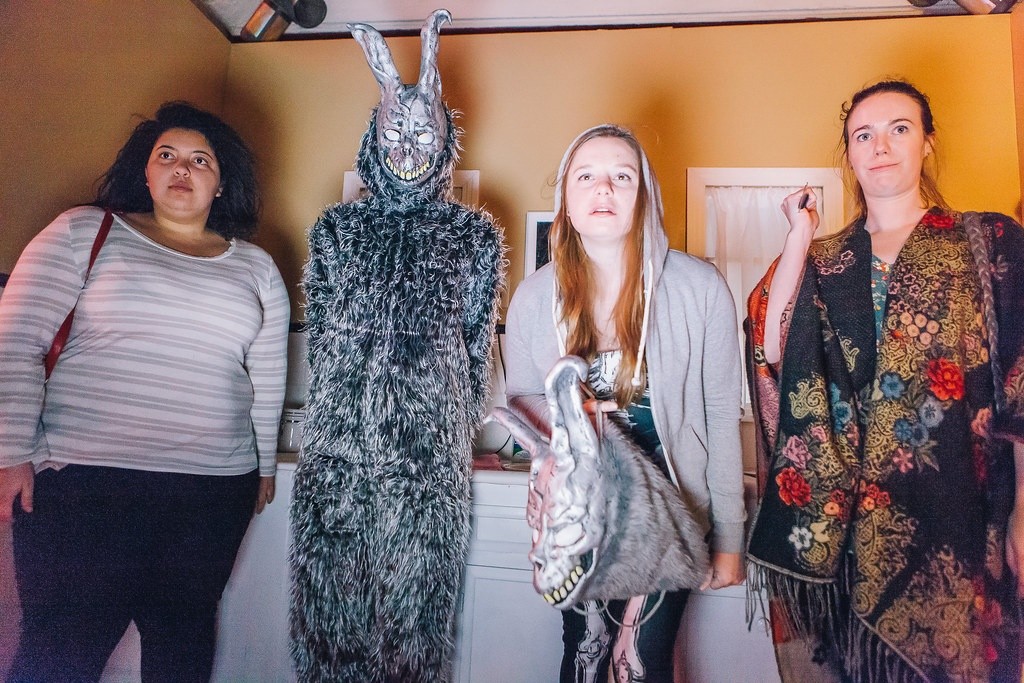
[524,212,554,279]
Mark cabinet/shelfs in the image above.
[209,462,782,683]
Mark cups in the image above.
[277,409,305,452]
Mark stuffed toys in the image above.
[292,9,507,683]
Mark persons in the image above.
[743,82,1023,683]
[0,102,291,683]
[504,123,748,683]
